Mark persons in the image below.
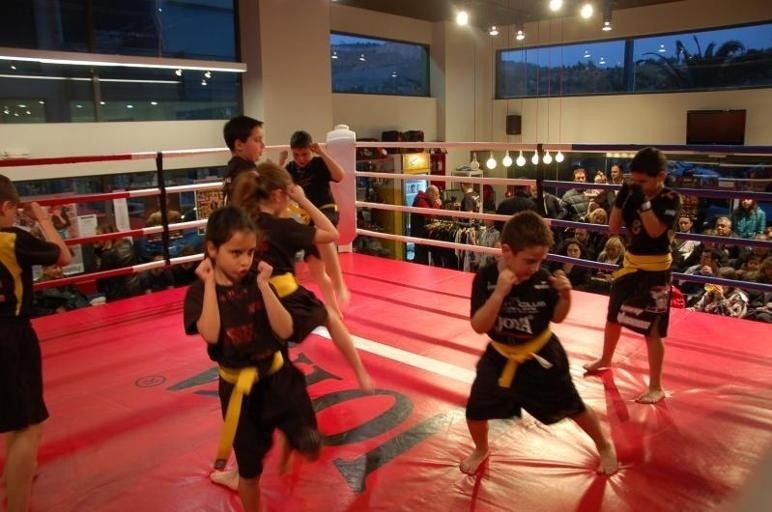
[0,173,74,509]
[279,131,352,322]
[223,113,273,189]
[579,146,680,403]
[410,165,772,324]
[457,211,619,478]
[181,204,324,511]
[205,162,377,491]
[28,205,206,324]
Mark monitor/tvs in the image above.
[685,108,747,145]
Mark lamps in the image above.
[470,0,563,170]
[601,0,615,32]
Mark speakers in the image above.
[506,115,522,135]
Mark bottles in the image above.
[703,283,717,293]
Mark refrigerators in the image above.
[376,152,431,261]
